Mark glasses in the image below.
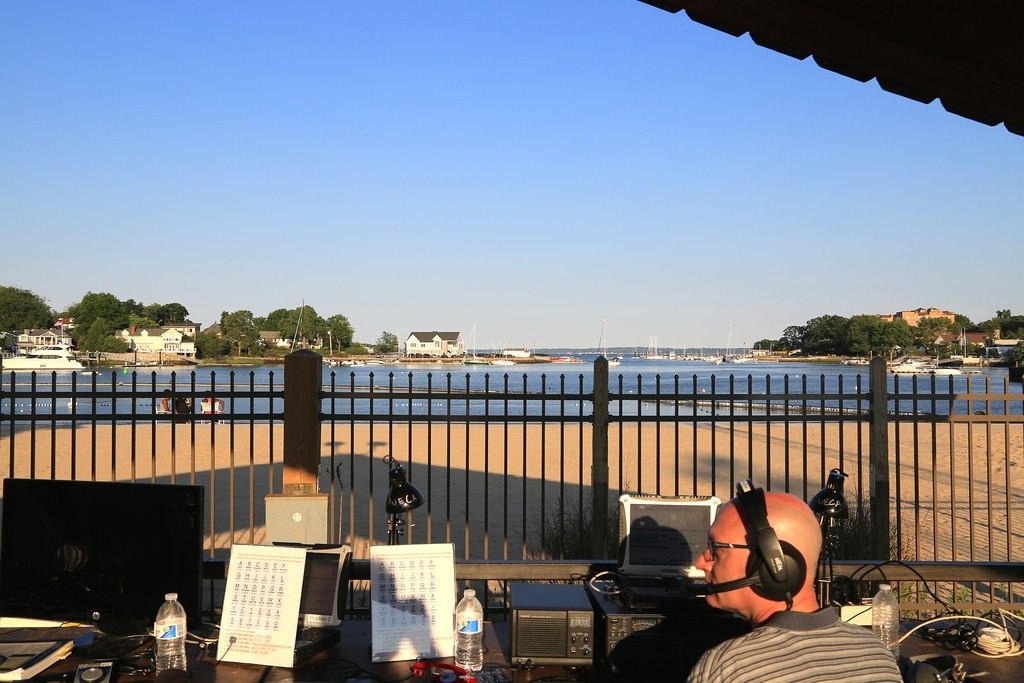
[707,540,758,560]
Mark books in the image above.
[0,640,75,682]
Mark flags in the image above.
[54,321,62,326]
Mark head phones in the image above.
[736,478,807,602]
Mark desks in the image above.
[0,610,1024,683]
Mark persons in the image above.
[685,477,910,683]
[158,389,172,414]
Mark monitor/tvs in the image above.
[1,477,204,631]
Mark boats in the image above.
[490,359,517,366]
[1,342,86,370]
[321,333,385,366]
[839,350,961,375]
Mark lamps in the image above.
[808,467,850,611]
[382,454,425,547]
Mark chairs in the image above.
[155,398,224,425]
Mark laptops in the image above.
[618,494,723,608]
[266,542,352,652]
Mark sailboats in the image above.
[462,323,490,365]
[631,324,759,364]
[597,320,620,365]
[949,327,980,364]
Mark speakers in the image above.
[509,582,594,664]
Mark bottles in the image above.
[872,584,900,660]
[456,589,483,671]
[155,593,188,677]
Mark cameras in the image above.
[72,661,116,683]
